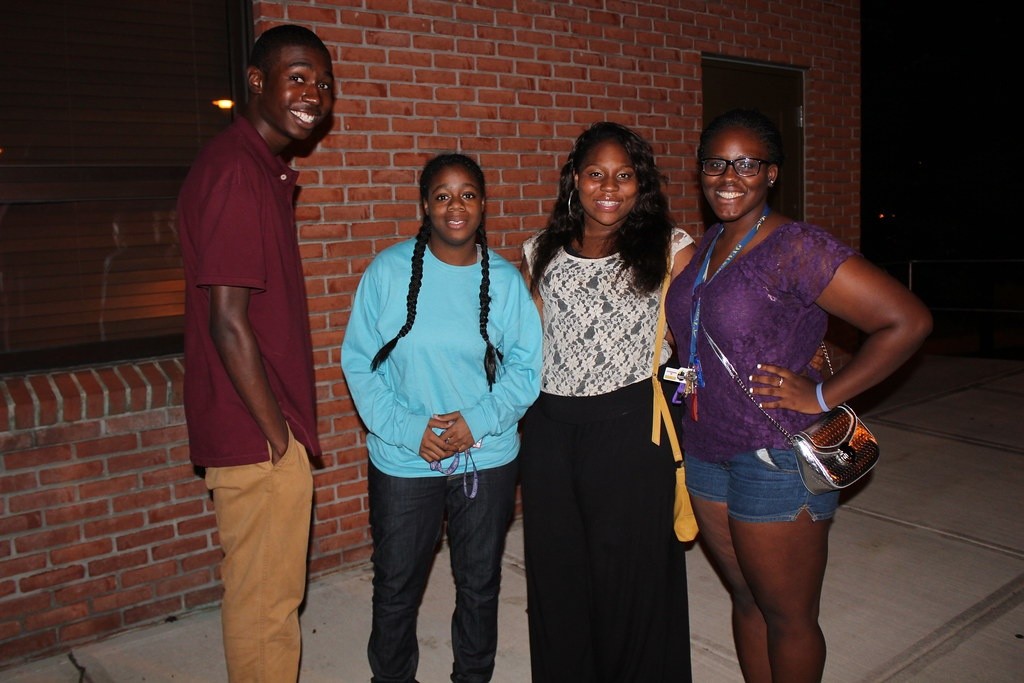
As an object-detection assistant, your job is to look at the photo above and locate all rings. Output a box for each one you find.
[779,377,784,386]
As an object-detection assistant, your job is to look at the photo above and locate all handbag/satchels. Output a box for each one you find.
[675,464,701,541]
[790,403,879,494]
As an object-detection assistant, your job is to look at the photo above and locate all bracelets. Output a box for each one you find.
[816,382,831,412]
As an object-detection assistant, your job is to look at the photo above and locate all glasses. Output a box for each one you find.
[701,156,772,176]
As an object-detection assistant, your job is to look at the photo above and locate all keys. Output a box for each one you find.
[684,371,698,394]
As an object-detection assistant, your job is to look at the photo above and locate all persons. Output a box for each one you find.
[177,25,337,683]
[519,121,833,683]
[341,154,544,683]
[664,111,933,683]
